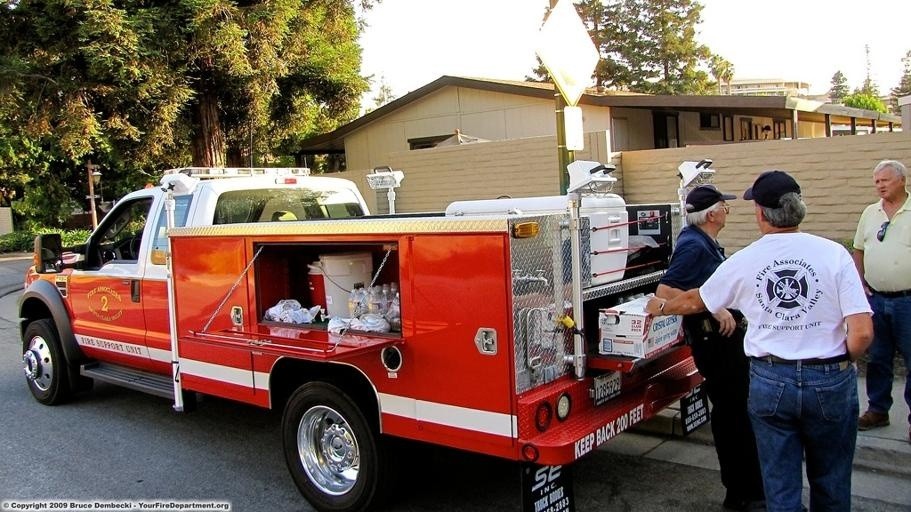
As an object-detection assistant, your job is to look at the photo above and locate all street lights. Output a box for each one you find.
[84,160,101,231]
[88,161,104,229]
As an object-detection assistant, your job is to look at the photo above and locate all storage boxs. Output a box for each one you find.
[598,296,683,359]
[446,193,629,285]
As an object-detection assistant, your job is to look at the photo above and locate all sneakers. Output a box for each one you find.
[857,409,890,430]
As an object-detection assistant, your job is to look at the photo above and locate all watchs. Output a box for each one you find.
[658,300,667,315]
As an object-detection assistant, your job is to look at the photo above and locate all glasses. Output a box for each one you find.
[877,221,890,241]
[716,203,730,214]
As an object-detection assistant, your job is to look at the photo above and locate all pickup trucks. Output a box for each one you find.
[17,156,720,512]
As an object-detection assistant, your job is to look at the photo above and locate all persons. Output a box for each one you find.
[656,185,765,511]
[646,171,874,511]
[853,160,910,443]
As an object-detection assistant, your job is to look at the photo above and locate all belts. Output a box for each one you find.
[869,285,911,299]
[750,354,851,366]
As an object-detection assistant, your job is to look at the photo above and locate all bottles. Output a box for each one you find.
[348,282,397,319]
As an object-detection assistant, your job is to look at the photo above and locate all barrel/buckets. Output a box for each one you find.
[319,252,374,323]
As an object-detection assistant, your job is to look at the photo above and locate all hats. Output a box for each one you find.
[686,185,737,214]
[743,170,801,209]
